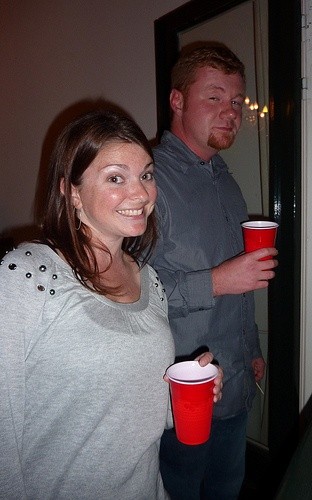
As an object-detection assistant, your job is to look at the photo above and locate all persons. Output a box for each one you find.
[0,112,224,500]
[126,40,278,500]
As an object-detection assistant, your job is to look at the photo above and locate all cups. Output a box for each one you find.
[242,221,279,281]
[166,361,219,446]
